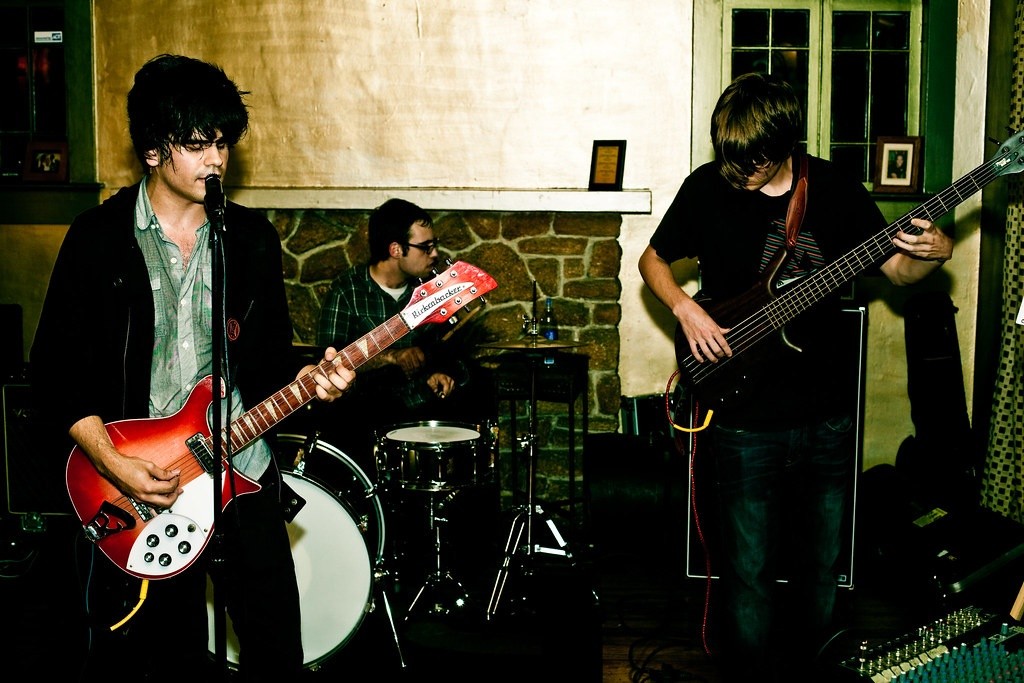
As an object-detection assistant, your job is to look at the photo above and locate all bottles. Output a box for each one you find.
[540,299,557,366]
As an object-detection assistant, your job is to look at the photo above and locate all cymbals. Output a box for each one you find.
[473,333,591,353]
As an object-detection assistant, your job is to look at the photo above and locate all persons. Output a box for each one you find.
[23,54,355,683]
[638,72,952,683]
[888,154,905,178]
[313,198,507,571]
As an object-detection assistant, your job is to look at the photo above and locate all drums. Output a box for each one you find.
[203,429,386,673]
[371,415,498,499]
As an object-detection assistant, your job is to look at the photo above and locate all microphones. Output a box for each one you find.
[205,173,225,233]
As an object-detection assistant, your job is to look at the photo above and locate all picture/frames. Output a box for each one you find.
[588,140,627,191]
[872,136,923,194]
[24,142,69,182]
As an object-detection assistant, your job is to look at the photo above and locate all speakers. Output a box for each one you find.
[684,306,868,585]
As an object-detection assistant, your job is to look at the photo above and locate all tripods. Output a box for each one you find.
[401,356,600,623]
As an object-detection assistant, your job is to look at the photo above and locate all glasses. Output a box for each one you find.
[408,237,437,255]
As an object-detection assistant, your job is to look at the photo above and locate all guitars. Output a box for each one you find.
[674,129,1024,433]
[61,258,501,584]
